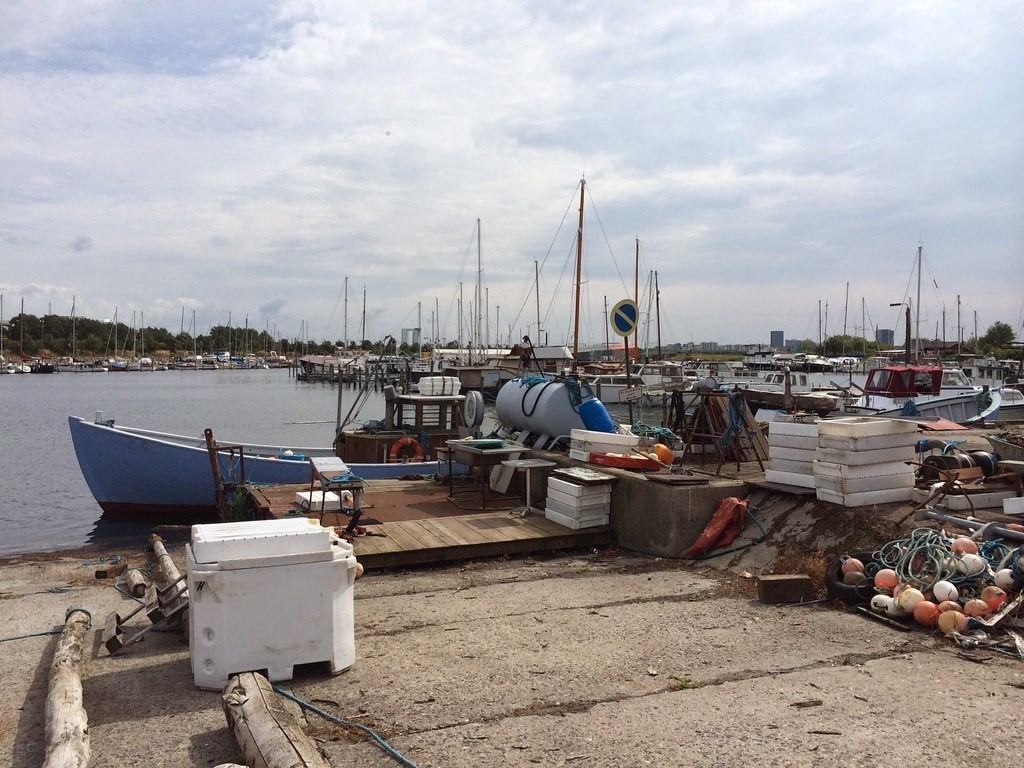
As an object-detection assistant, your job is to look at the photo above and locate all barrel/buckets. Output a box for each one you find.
[279,447,304,461]
[579,398,615,432]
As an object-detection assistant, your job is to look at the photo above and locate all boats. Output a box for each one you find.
[68,333,768,515]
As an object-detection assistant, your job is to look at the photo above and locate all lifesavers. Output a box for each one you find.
[389,438,423,463]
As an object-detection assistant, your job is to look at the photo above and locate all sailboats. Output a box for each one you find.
[298,170,1023,421]
[0,294,297,374]
